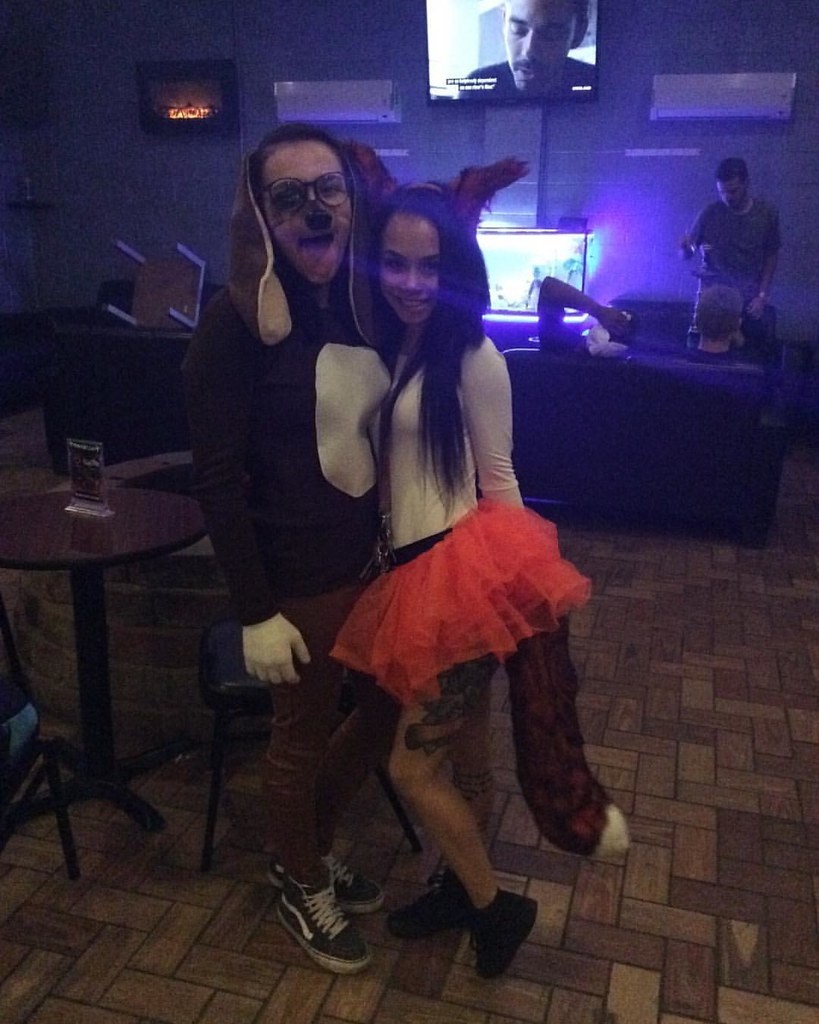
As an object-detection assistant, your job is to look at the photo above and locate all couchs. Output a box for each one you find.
[499,346,785,549]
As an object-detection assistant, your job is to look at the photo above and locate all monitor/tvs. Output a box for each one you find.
[423,0,600,107]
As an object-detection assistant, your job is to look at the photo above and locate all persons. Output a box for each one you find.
[534,276,642,357]
[330,179,541,979]
[676,155,782,349]
[670,282,751,366]
[456,0,596,101]
[181,122,397,979]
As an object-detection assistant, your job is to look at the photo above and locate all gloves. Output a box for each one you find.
[241,611,311,687]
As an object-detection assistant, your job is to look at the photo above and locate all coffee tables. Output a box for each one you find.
[0,485,207,836]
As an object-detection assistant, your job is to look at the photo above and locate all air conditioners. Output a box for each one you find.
[272,78,402,126]
[648,72,799,123]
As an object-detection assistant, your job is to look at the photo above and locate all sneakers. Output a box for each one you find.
[265,850,386,914]
[386,870,478,941]
[476,885,538,979]
[276,869,371,975]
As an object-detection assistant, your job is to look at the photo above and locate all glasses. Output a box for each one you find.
[259,170,352,213]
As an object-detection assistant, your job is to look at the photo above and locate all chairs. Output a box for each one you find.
[198,612,424,878]
[0,591,167,879]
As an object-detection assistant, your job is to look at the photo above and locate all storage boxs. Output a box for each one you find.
[476,216,594,326]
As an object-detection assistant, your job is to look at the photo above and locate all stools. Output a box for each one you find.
[98,233,207,334]
